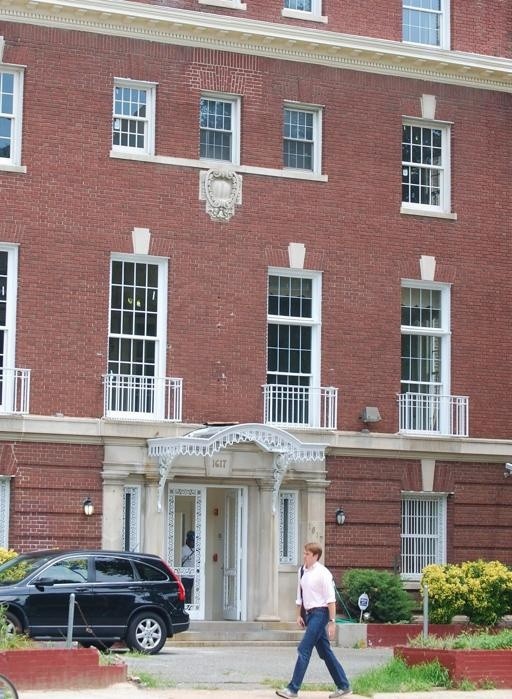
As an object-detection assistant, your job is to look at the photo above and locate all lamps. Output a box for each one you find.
[336,504,347,526]
[83,496,95,517]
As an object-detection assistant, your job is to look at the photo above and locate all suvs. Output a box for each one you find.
[1,547,192,657]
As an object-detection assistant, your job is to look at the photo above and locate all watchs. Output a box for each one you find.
[328,618,335,623]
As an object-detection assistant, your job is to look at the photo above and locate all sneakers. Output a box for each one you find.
[329,689,352,699]
[276,688,298,699]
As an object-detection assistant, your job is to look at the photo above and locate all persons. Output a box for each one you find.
[180,531,195,604]
[275,541,353,698]
